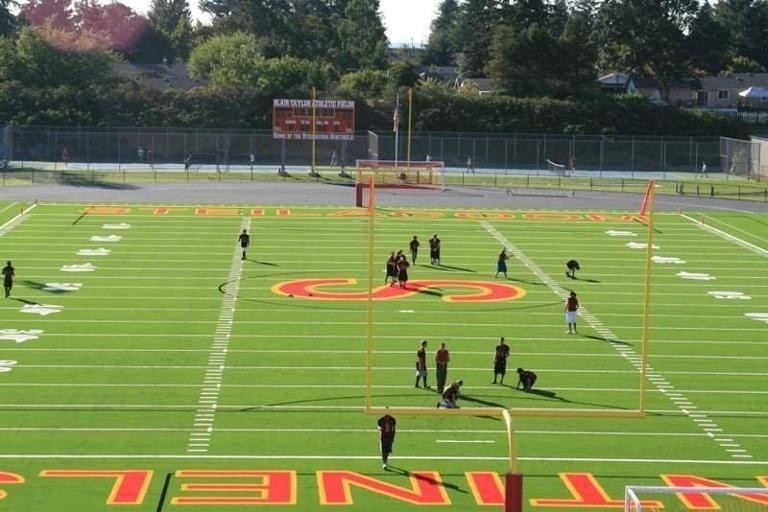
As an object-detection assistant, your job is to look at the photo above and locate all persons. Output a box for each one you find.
[377,409,395,470]
[409,235,419,265]
[238,230,249,261]
[492,337,511,386]
[414,340,429,387]
[700,161,709,177]
[428,234,439,265]
[433,342,451,393]
[425,153,432,169]
[565,292,579,334]
[568,155,576,174]
[436,378,464,408]
[565,259,581,278]
[493,250,513,278]
[249,150,255,169]
[464,156,473,174]
[383,250,409,289]
[2,260,15,298]
[330,151,336,167]
[516,368,538,391]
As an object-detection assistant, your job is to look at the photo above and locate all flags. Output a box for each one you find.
[392,97,398,136]
